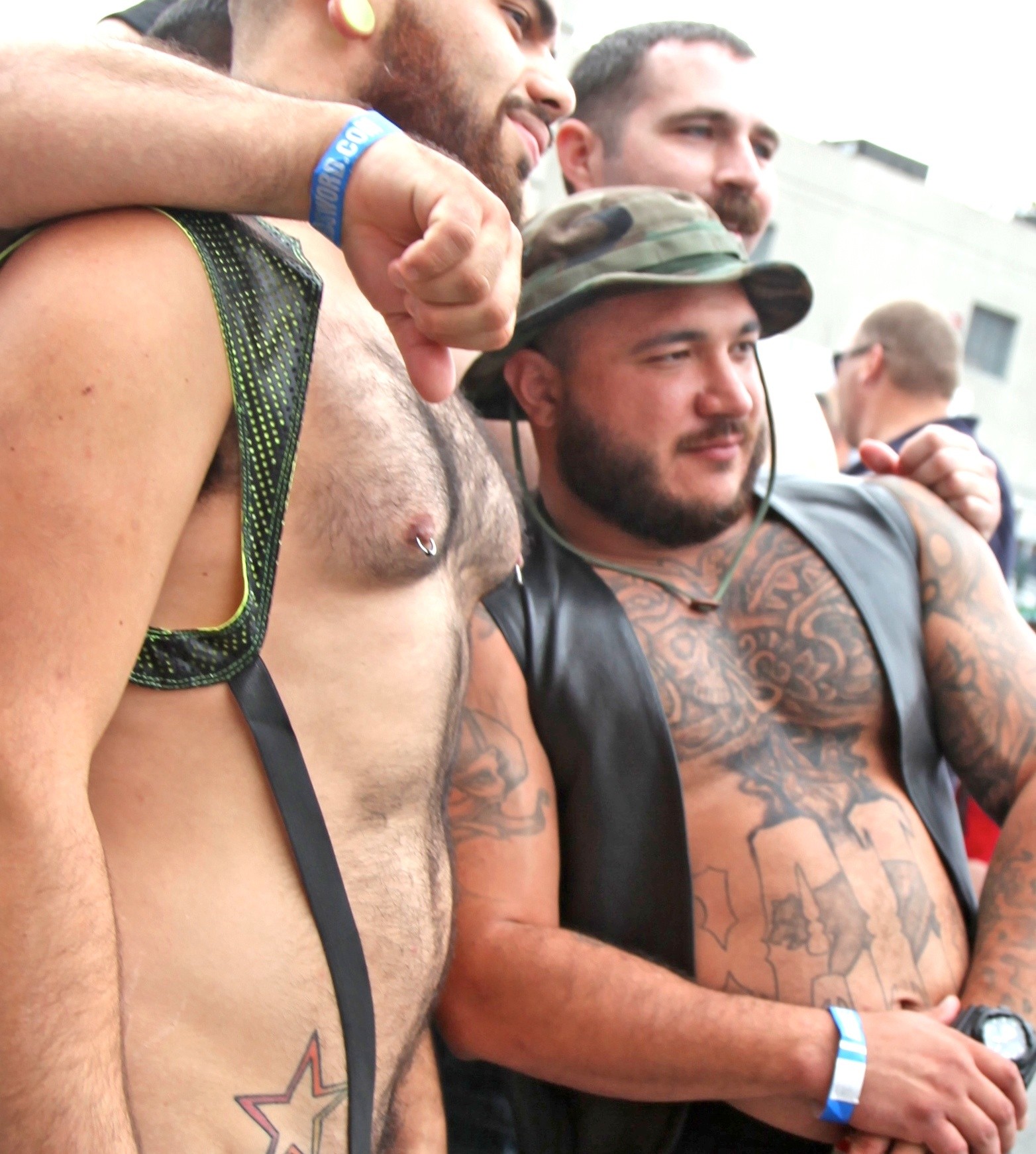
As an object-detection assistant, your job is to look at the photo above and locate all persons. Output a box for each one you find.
[0,0,1036,1154]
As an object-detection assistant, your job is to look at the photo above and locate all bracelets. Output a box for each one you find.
[308,107,399,251]
[818,1005,867,1125]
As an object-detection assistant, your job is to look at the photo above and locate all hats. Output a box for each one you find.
[459,186,813,421]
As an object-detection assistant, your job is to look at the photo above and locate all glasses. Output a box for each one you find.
[832,340,886,377]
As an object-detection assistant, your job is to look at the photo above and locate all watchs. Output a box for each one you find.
[948,1004,1036,1089]
